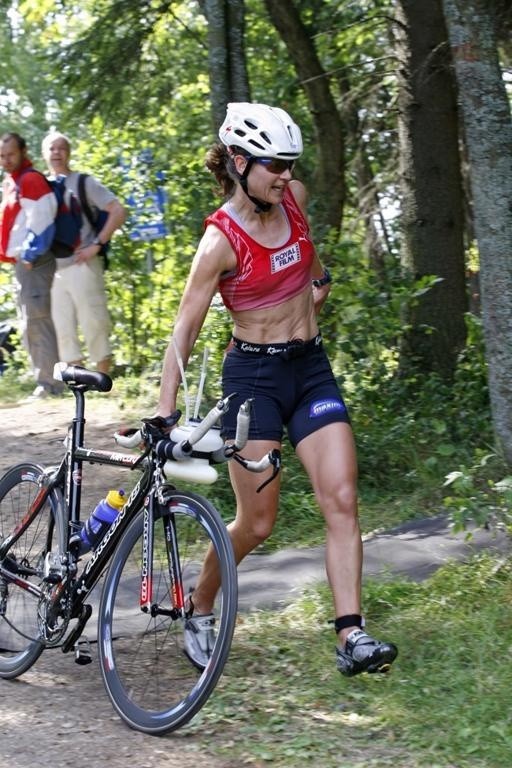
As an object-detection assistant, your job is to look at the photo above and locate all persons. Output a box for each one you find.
[0,131,58,398]
[40,131,127,388]
[149,102,399,678]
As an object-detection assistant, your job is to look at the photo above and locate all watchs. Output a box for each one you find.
[91,238,103,246]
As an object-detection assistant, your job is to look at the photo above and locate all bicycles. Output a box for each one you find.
[0,361,284,737]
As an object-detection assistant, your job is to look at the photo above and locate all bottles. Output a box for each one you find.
[79,489,129,555]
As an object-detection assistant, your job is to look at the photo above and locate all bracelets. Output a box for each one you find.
[313,266,331,287]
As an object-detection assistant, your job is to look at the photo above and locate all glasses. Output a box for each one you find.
[244,154,295,175]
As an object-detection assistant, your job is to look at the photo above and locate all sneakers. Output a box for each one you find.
[181,606,221,674]
[334,629,400,679]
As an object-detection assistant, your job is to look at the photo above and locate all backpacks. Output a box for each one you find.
[78,173,113,256]
[17,168,86,258]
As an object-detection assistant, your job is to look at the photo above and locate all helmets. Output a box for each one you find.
[217,101,305,161]
